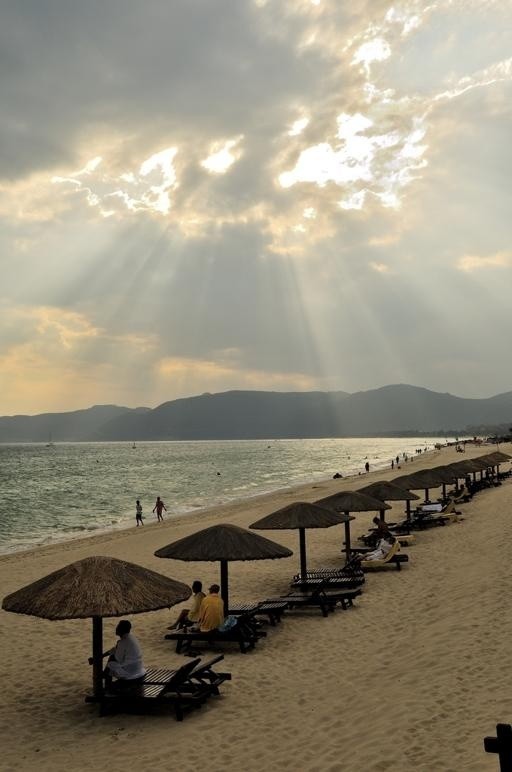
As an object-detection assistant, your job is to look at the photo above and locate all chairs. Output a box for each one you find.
[260,553,409,617]
[86,652,233,718]
[162,603,287,654]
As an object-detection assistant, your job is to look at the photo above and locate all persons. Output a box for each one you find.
[152,497,167,523]
[102,620,146,689]
[365,462,369,472]
[365,517,389,543]
[333,473,343,479]
[364,535,396,562]
[453,484,464,498]
[488,469,494,485]
[166,580,206,630]
[197,584,224,632]
[358,532,392,557]
[135,500,144,526]
